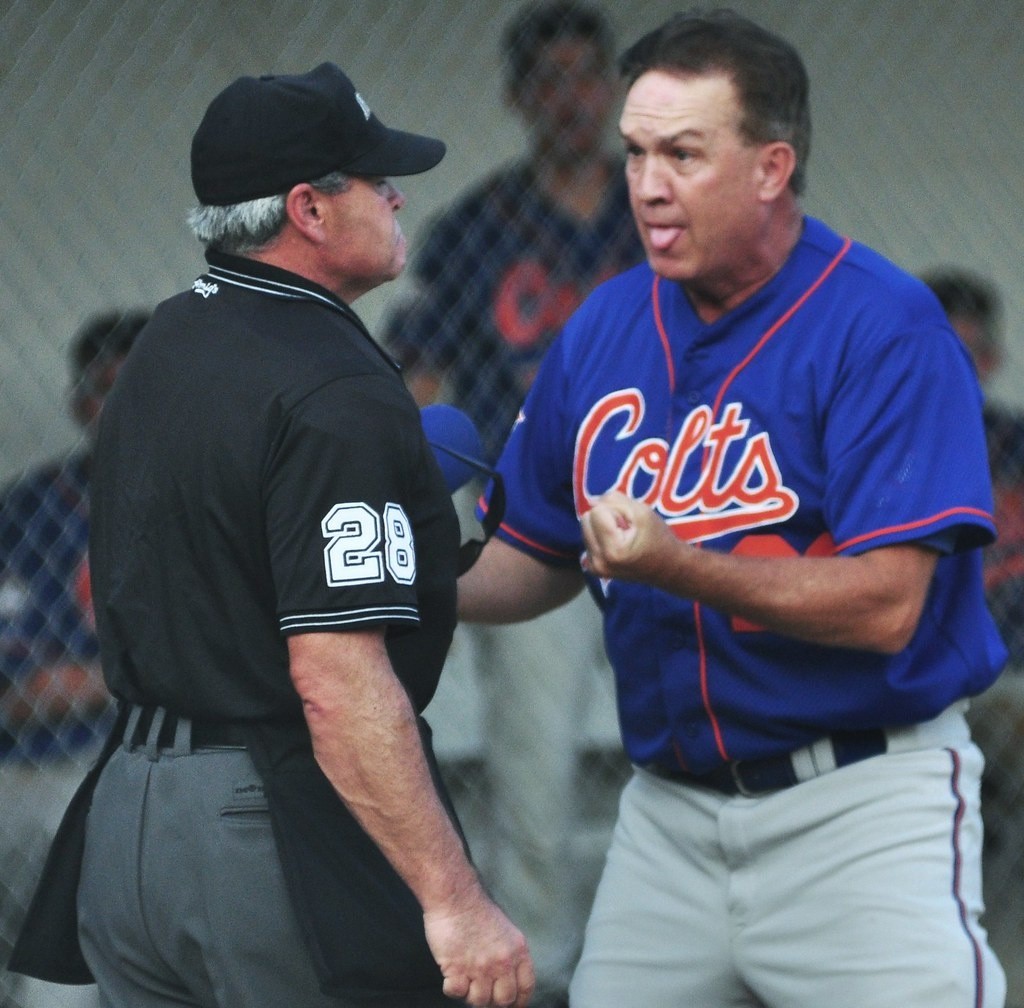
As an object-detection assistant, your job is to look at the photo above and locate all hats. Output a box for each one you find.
[189,62,446,207]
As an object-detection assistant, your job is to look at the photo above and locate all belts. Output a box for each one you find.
[646,729,889,797]
[137,713,250,748]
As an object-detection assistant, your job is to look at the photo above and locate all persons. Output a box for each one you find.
[918,266,1024,1008]
[7,58,535,1008]
[367,0,653,1008]
[0,309,154,1008]
[457,9,1008,1008]
